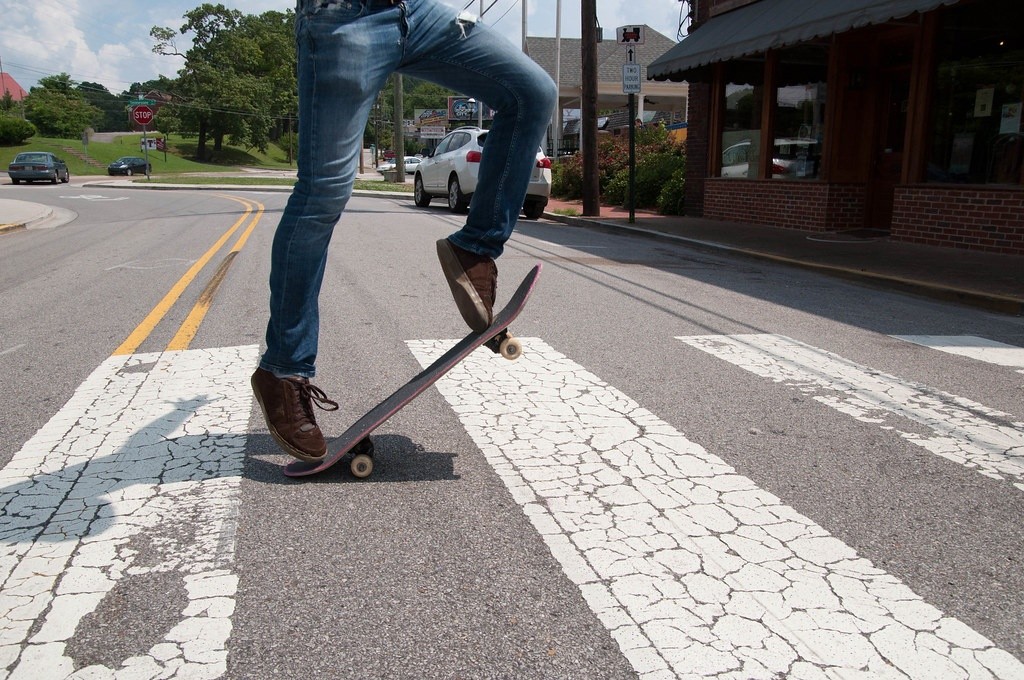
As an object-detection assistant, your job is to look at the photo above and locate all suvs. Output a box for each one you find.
[410,126,553,220]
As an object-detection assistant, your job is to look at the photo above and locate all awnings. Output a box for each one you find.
[647,0,963,84]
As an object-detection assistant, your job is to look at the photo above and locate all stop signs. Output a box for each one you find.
[132,104,155,125]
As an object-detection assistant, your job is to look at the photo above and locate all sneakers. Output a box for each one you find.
[251,367,338,461]
[436,236,498,332]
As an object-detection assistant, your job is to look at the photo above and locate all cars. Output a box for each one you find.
[383,149,399,160]
[7,152,70,185]
[108,156,152,177]
[375,156,424,175]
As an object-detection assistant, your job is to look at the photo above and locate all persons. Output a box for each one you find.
[249,0,558,461]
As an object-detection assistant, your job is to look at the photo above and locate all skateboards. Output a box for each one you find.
[283,264,542,478]
